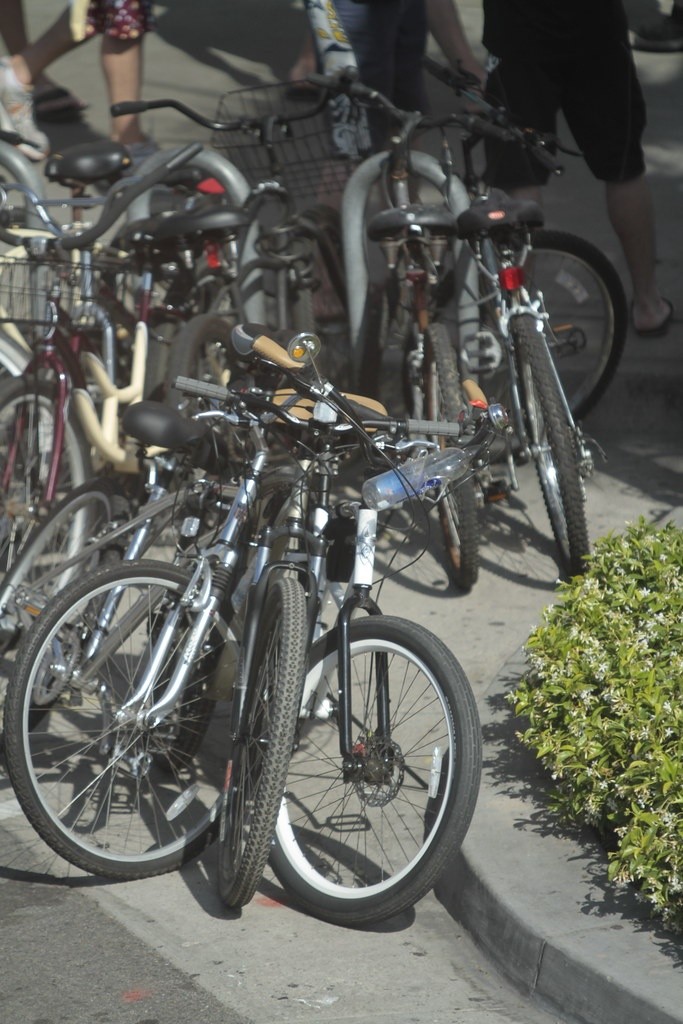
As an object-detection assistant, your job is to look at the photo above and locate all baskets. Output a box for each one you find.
[0,254,129,330]
[208,80,369,196]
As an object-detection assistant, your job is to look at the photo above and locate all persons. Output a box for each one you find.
[292,0,490,316]
[0,0,162,174]
[482,1,677,337]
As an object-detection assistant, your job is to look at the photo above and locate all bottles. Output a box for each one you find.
[362,444,481,512]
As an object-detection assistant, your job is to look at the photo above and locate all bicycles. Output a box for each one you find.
[0,39,622,931]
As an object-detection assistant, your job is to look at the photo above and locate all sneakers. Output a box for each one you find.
[129,139,189,192]
[1,56,50,162]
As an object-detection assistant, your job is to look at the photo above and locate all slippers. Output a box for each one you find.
[632,298,673,335]
[35,88,83,123]
